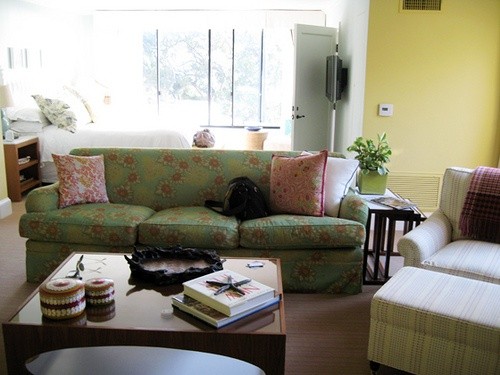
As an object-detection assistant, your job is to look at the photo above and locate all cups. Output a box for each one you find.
[5,130,14,142]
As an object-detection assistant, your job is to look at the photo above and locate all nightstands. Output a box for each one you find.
[3,136,41,202]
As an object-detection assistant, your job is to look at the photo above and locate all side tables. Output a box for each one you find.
[361,194,426,285]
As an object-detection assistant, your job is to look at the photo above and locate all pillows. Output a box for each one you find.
[301,151,360,217]
[270,150,328,217]
[51,153,109,209]
[32,90,94,134]
[10,121,42,133]
[4,96,48,123]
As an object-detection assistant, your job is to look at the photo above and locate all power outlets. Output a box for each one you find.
[379,104,392,116]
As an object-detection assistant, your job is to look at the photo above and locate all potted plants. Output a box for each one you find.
[347,132,392,195]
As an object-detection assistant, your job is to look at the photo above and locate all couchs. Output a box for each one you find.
[19,149,369,294]
[367,167,499,375]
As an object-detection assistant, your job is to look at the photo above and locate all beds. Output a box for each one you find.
[8,80,191,184]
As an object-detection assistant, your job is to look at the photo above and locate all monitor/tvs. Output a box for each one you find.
[326,55,347,103]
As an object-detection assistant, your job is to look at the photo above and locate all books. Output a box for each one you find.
[170,289,283,329]
[371,195,418,211]
[182,268,278,318]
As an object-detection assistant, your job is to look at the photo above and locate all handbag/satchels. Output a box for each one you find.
[204,176,270,219]
